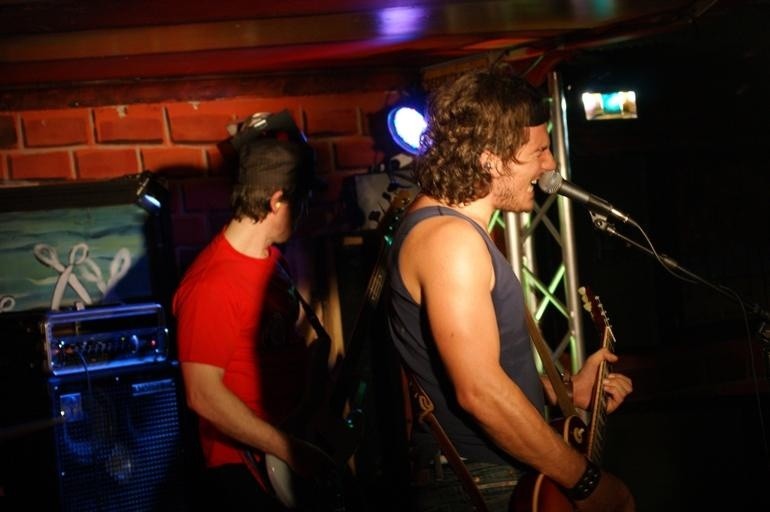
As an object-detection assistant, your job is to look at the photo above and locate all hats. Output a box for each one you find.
[234,134,313,185]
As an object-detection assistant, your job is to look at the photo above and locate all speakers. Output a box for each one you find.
[44,362,188,512]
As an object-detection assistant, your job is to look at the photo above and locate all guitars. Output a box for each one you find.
[258,187,421,511]
[517,292,640,512]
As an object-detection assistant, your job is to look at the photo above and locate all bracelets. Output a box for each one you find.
[555,377,574,408]
[559,455,602,500]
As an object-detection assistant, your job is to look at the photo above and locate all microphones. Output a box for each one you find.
[537,167,629,225]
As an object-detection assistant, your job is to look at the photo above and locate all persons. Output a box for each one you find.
[167,140,331,511]
[380,66,637,511]
